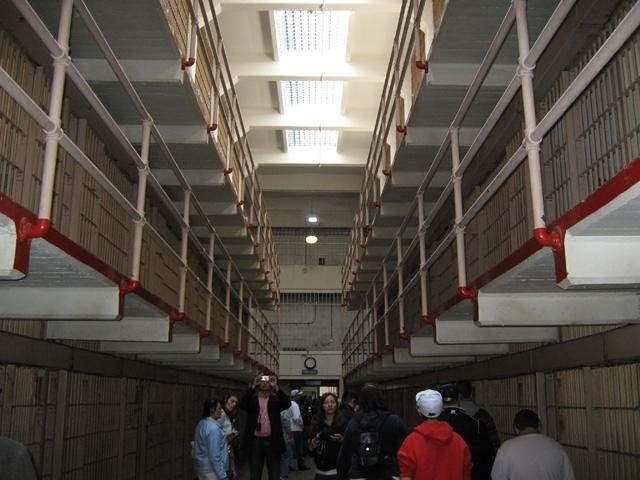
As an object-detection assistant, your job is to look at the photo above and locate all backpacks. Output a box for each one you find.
[348,411,393,480]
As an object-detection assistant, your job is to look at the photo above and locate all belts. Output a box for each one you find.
[252,436,270,440]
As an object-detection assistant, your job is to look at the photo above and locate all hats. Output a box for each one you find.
[291,390,303,396]
[437,382,459,403]
[415,389,444,418]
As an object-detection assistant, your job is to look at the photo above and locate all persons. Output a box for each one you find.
[490,409,571,477]
[194,374,500,478]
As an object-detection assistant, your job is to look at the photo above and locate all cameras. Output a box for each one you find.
[260,375,269,382]
[319,431,329,441]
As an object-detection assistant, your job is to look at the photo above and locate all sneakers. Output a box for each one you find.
[301,467,311,471]
